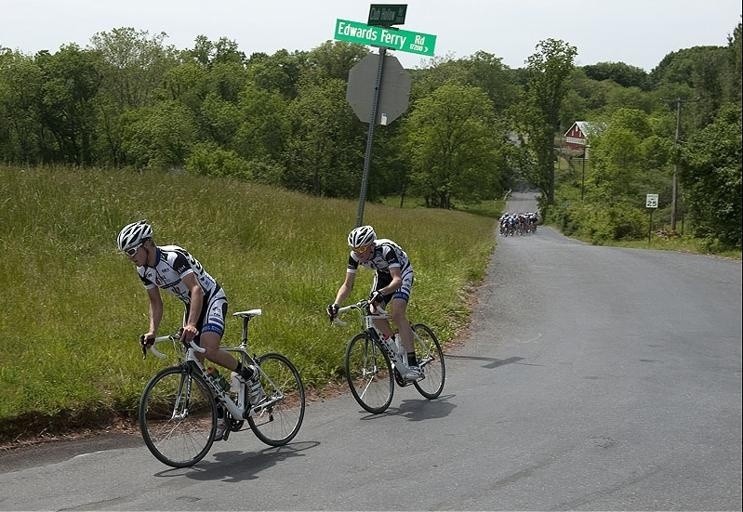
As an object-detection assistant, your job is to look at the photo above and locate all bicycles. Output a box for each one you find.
[326,292,447,415]
[499,219,537,237]
[136,307,305,468]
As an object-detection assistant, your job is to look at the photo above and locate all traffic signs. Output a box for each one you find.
[334,18,436,57]
[646,194,659,207]
[367,3,407,26]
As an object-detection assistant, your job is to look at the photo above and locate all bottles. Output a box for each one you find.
[206,366,233,394]
[382,334,398,354]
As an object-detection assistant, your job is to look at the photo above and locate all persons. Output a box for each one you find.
[326,225,422,379]
[498,210,540,237]
[116,220,262,442]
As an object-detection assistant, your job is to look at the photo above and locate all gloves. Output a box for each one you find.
[326,304,339,319]
[366,289,385,307]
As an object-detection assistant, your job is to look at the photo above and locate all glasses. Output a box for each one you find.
[125,238,149,258]
[351,245,373,255]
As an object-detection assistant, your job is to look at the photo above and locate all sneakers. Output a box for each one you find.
[242,364,264,406]
[207,411,232,441]
[404,364,423,381]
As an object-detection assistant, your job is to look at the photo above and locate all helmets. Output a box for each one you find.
[503,211,539,226]
[346,225,377,248]
[116,219,154,252]
